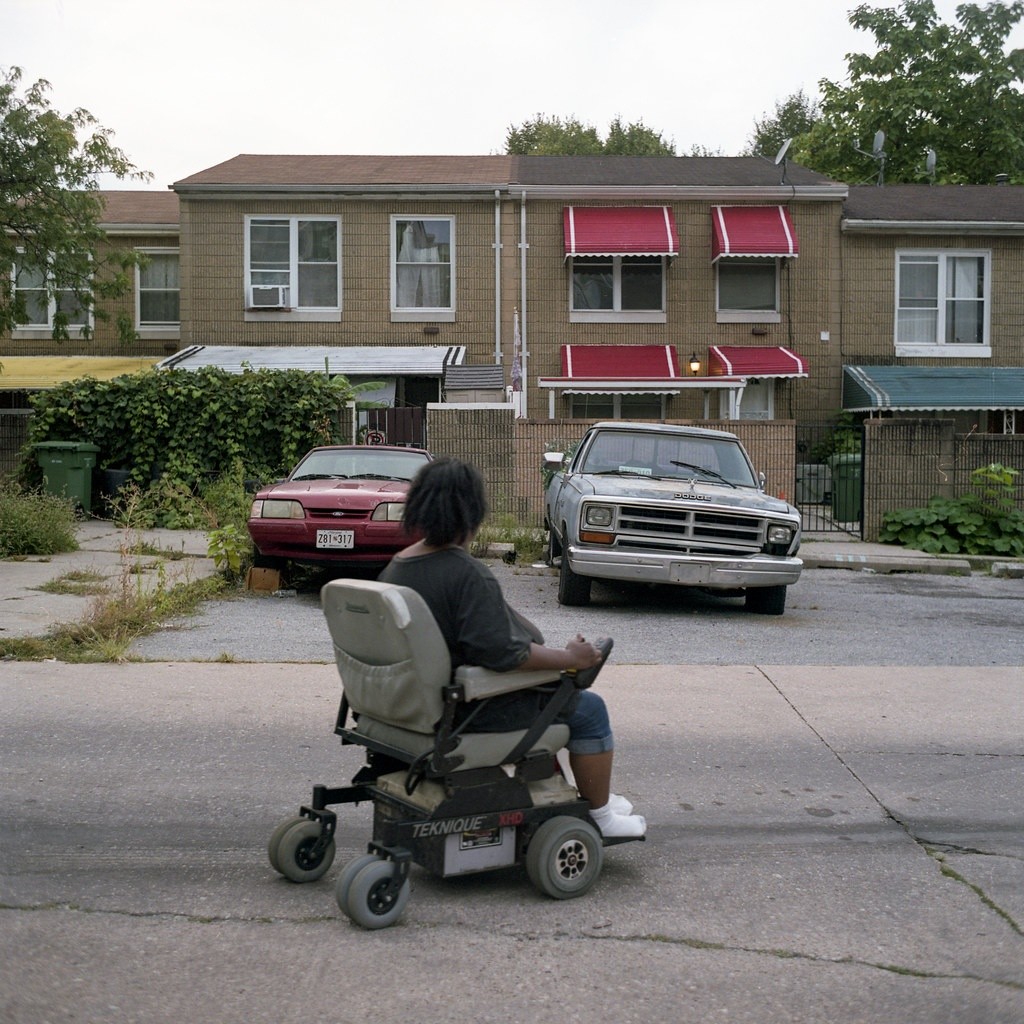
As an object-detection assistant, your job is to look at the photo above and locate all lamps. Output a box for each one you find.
[690,352,700,376]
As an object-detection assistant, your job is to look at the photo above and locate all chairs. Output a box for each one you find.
[320,578,580,772]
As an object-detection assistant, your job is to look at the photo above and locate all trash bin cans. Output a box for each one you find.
[32,440,101,522]
[827,453,861,523]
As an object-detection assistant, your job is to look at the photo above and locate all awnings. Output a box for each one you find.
[559,343,681,395]
[708,346,808,379]
[710,205,799,264]
[156,345,467,375]
[563,205,679,264]
[841,365,1024,411]
[0,356,169,394]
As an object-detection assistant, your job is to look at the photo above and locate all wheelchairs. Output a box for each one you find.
[266,579,651,934]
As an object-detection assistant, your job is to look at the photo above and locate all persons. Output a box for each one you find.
[377,458,647,836]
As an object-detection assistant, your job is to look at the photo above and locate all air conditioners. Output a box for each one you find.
[251,285,286,308]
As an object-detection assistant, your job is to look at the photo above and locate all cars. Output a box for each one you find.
[541,423,803,618]
[245,447,441,595]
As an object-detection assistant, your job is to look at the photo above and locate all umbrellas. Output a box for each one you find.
[510,306,522,391]
